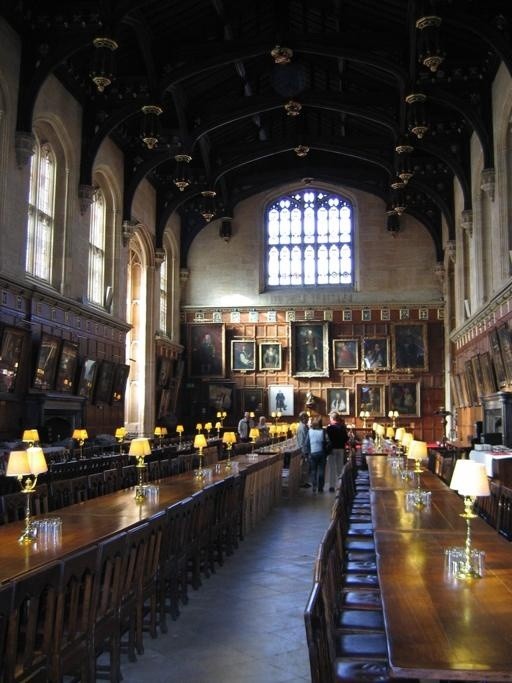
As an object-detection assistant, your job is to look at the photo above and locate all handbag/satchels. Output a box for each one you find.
[322,429,333,456]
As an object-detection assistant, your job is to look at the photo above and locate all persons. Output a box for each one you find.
[304,415,333,496]
[336,416,346,433]
[296,411,312,487]
[326,412,348,492]
[156,360,175,393]
[236,411,256,442]
[257,415,270,441]
[190,323,426,418]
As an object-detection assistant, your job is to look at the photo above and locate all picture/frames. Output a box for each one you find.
[0,321,132,409]
[156,317,431,424]
[442,321,512,409]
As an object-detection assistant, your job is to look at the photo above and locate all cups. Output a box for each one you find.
[445,546,487,577]
[32,515,63,532]
[144,484,160,495]
[403,488,432,504]
